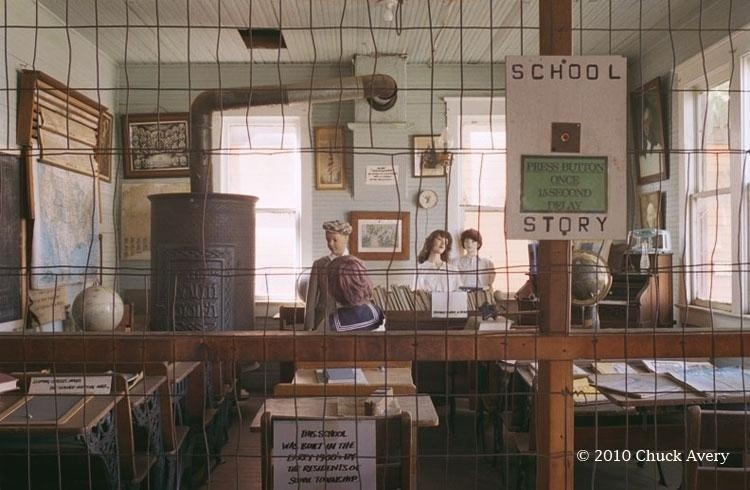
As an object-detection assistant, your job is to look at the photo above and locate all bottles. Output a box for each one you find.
[639,242,651,272]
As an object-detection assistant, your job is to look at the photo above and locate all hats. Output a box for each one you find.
[324,221,352,235]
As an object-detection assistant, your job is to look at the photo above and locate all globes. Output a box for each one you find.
[72,285,124,332]
[571,250,613,329]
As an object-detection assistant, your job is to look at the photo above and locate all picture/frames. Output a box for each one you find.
[639,191,663,229]
[121,112,189,179]
[631,75,668,184]
[313,126,346,190]
[351,210,410,260]
[412,134,447,177]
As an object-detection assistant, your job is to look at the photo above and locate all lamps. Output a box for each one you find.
[378,0,398,21]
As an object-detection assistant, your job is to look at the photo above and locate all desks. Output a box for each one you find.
[278,304,541,330]
[250,359,439,490]
[452,360,750,490]
[0,362,233,490]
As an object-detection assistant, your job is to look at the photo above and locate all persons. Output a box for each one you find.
[411,228,463,292]
[635,105,665,177]
[312,254,387,333]
[454,227,497,288]
[302,219,366,332]
[643,198,661,229]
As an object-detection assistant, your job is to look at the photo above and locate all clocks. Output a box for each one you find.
[419,189,438,208]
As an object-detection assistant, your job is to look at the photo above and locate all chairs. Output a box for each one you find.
[63,302,134,332]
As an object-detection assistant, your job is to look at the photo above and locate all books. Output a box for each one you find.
[368,283,516,332]
[0,371,21,395]
[315,367,369,384]
[325,367,355,383]
[502,357,749,409]
[335,396,402,417]
[127,369,144,391]
[106,368,139,382]
